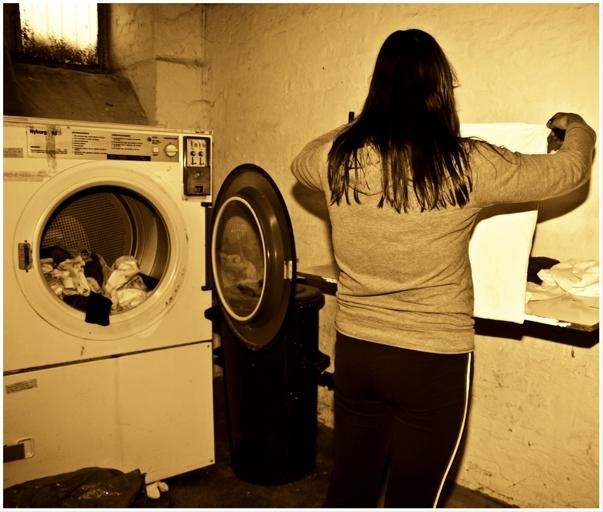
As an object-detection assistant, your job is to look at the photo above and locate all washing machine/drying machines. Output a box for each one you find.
[3,117,300,490]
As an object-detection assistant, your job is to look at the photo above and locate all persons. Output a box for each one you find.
[290,28,596,508]
